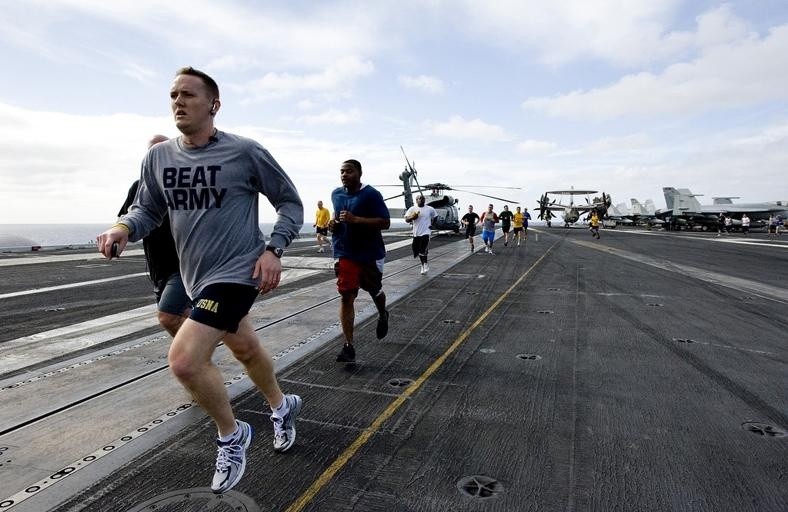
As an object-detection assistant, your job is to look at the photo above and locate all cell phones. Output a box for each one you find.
[112,242,119,257]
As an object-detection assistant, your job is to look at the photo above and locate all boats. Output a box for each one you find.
[1,218,788,512]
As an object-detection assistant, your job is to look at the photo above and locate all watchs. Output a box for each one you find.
[264,243,285,260]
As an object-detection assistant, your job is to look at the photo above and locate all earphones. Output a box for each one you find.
[212,104,216,110]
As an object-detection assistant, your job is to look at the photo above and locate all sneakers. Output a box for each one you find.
[210,419,251,494]
[592,232,600,239]
[485,246,492,254]
[336,343,355,362]
[330,242,333,249]
[421,263,429,274]
[470,238,474,252]
[316,248,324,252]
[270,394,302,451]
[377,311,388,338]
[504,236,527,247]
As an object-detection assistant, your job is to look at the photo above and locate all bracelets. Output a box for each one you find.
[113,223,131,235]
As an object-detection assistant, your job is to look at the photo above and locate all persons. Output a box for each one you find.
[461,205,481,252]
[313,200,333,253]
[480,203,500,256]
[511,207,524,248]
[646,212,786,237]
[328,160,392,364]
[98,65,302,496]
[522,208,531,235]
[404,194,439,276]
[589,210,600,239]
[497,205,513,245]
[121,135,192,339]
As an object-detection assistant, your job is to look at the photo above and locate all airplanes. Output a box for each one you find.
[532,186,610,227]
[603,186,787,231]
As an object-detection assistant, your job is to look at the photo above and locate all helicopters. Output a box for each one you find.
[370,142,522,235]
